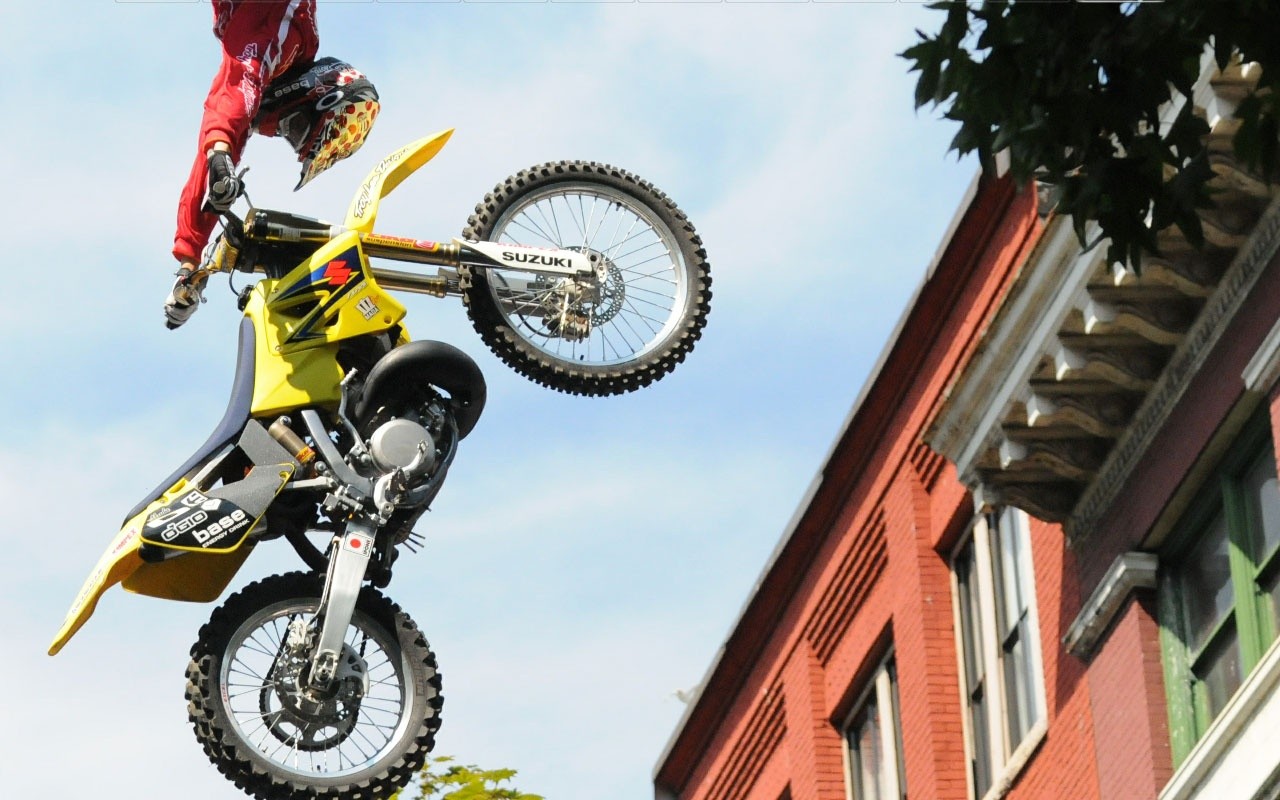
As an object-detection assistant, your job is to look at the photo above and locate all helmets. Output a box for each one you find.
[253,56,381,192]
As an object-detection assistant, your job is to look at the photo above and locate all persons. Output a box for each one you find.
[165,1,380,332]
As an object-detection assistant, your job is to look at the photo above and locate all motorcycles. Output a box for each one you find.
[47,125,711,800]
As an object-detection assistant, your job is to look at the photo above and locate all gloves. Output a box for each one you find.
[200,150,242,217]
[163,266,200,328]
